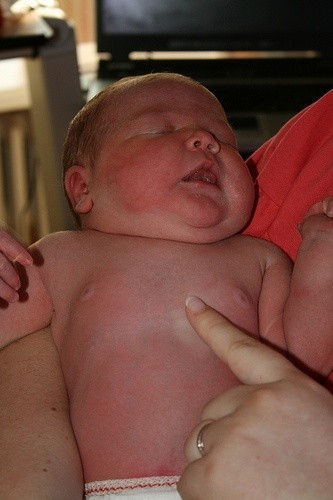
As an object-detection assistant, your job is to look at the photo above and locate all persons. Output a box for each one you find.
[0,72,333,500]
[0,87,333,500]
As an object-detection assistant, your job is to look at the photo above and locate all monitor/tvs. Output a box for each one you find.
[94,0,333,81]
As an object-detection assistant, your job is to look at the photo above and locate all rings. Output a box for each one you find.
[197,421,213,457]
